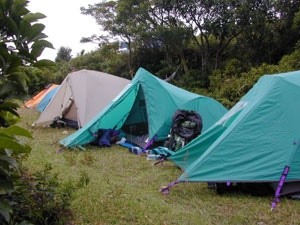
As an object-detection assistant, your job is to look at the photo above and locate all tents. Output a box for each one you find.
[55,66,229,154]
[154,67,300,214]
[26,69,131,131]
[23,84,61,113]
